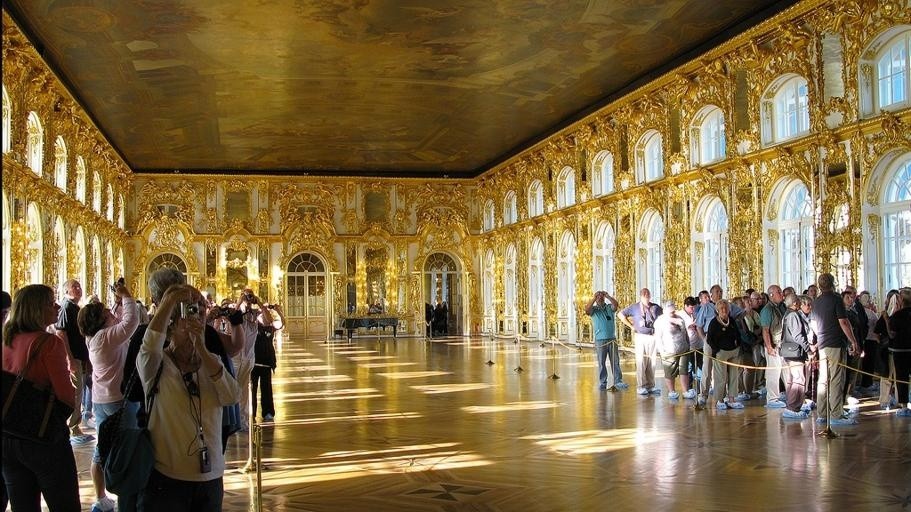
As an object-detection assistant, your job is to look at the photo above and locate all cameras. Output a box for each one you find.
[268,305,274,309]
[246,292,252,300]
[220,308,230,315]
[181,299,202,318]
[109,278,127,291]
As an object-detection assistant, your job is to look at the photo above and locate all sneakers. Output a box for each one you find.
[696,395,706,405]
[716,401,743,409]
[852,382,879,397]
[816,410,855,425]
[91,498,118,511]
[767,391,786,407]
[599,384,606,390]
[668,389,695,399]
[734,388,766,400]
[638,386,660,394]
[782,400,815,418]
[70,433,92,444]
[614,383,628,389]
[881,394,911,416]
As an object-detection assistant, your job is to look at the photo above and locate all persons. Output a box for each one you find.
[425,301,449,335]
[585,272,911,424]
[2,269,286,512]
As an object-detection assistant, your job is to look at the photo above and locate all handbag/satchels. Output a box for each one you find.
[99,409,155,496]
[0,369,74,445]
[779,342,801,359]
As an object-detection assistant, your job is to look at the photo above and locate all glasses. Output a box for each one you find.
[182,371,201,398]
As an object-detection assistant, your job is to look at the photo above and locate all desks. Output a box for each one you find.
[340,315,398,338]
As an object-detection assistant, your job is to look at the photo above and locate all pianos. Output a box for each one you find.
[340,318,399,345]
